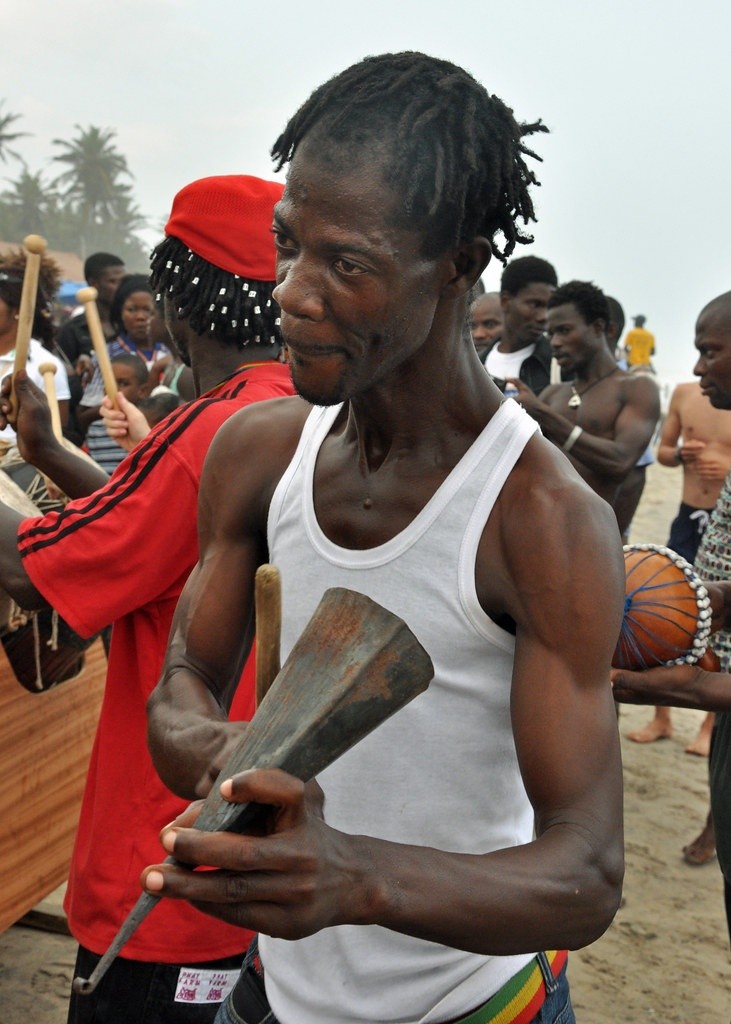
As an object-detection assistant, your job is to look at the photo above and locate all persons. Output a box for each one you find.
[0,244,728,947]
[141,47,625,1024]
[0,173,284,1024]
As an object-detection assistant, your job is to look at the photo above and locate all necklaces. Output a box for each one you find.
[567,366,621,410]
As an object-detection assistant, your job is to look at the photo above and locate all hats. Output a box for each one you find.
[164,175,285,282]
[633,315,646,322]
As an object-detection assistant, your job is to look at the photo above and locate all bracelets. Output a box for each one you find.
[675,445,684,465]
